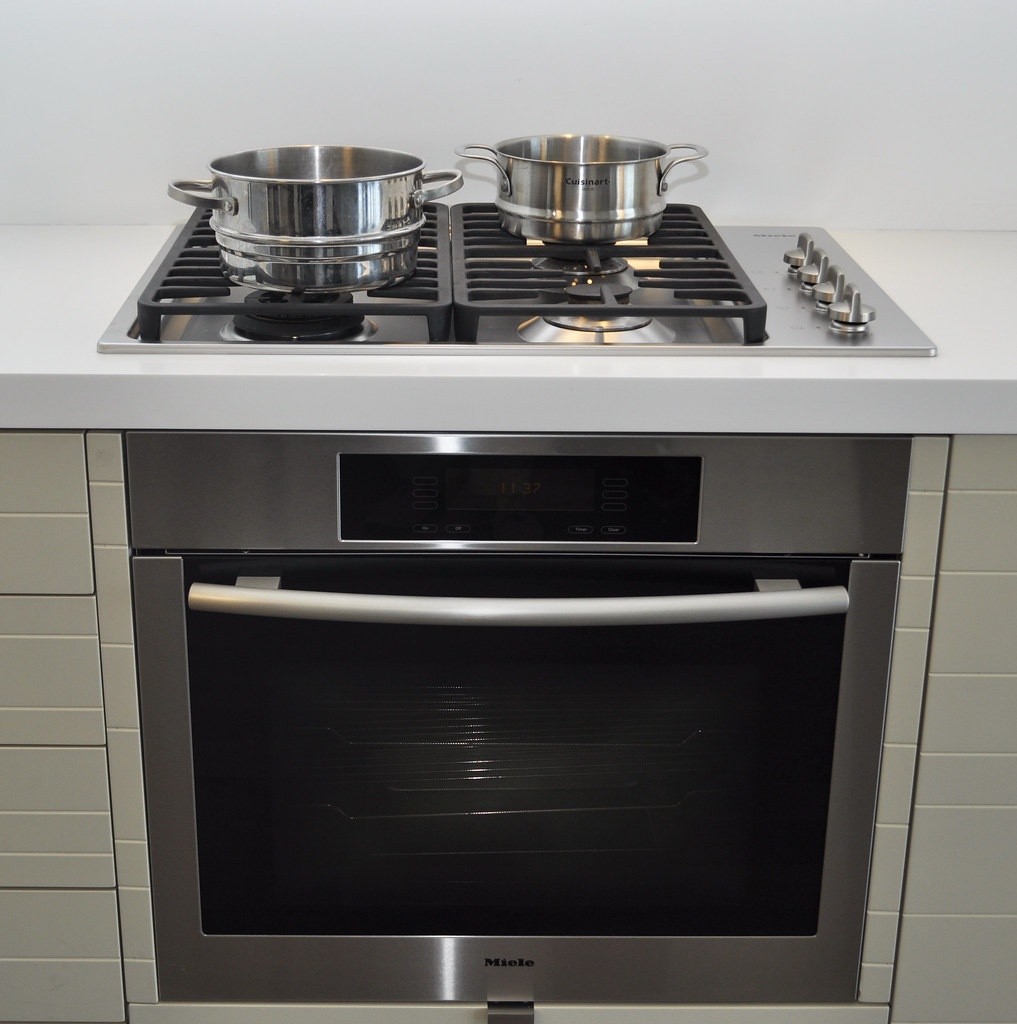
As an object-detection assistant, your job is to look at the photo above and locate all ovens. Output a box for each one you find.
[124,429,914,1024]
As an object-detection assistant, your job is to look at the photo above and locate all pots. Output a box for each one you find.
[166,145,465,294]
[454,134,708,244]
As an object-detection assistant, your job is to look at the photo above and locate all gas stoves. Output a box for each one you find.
[96,203,937,358]
